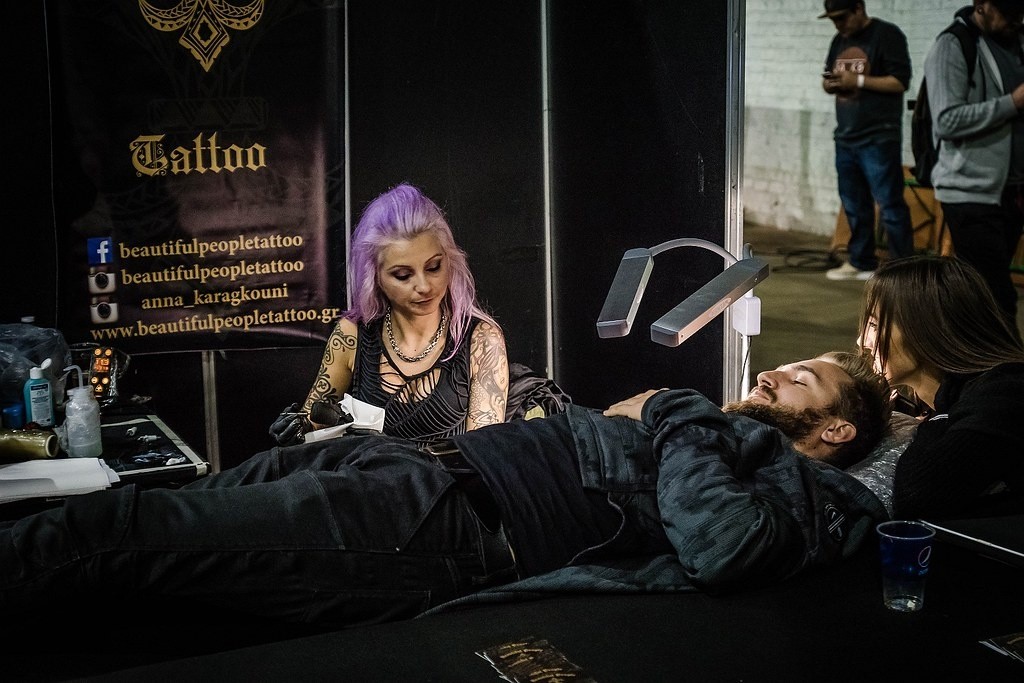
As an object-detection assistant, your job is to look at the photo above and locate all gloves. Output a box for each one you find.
[268,403,314,447]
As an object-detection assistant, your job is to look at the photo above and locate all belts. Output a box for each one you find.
[422,438,518,589]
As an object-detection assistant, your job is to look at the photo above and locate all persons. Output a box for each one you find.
[816,0,915,283]
[853,252,1024,525]
[0,350,895,667]
[267,183,511,450]
[928,0,1023,327]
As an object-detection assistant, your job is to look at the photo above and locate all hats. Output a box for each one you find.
[816,0,864,19]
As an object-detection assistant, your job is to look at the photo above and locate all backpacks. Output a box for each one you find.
[906,20,977,185]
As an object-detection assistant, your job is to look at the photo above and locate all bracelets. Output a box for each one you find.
[856,74,866,89]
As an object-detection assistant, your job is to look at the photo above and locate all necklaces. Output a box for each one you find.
[384,304,447,361]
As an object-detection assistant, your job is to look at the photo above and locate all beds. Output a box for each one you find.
[99,406,964,682]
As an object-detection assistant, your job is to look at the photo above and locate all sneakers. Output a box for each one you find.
[825,260,875,282]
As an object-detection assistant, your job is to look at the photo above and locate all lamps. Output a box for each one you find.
[595,236,772,404]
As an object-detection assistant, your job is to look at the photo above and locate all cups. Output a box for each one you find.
[876,519,936,612]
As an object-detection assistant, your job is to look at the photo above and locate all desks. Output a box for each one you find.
[0,411,211,502]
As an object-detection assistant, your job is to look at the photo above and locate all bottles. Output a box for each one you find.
[67,385,103,458]
